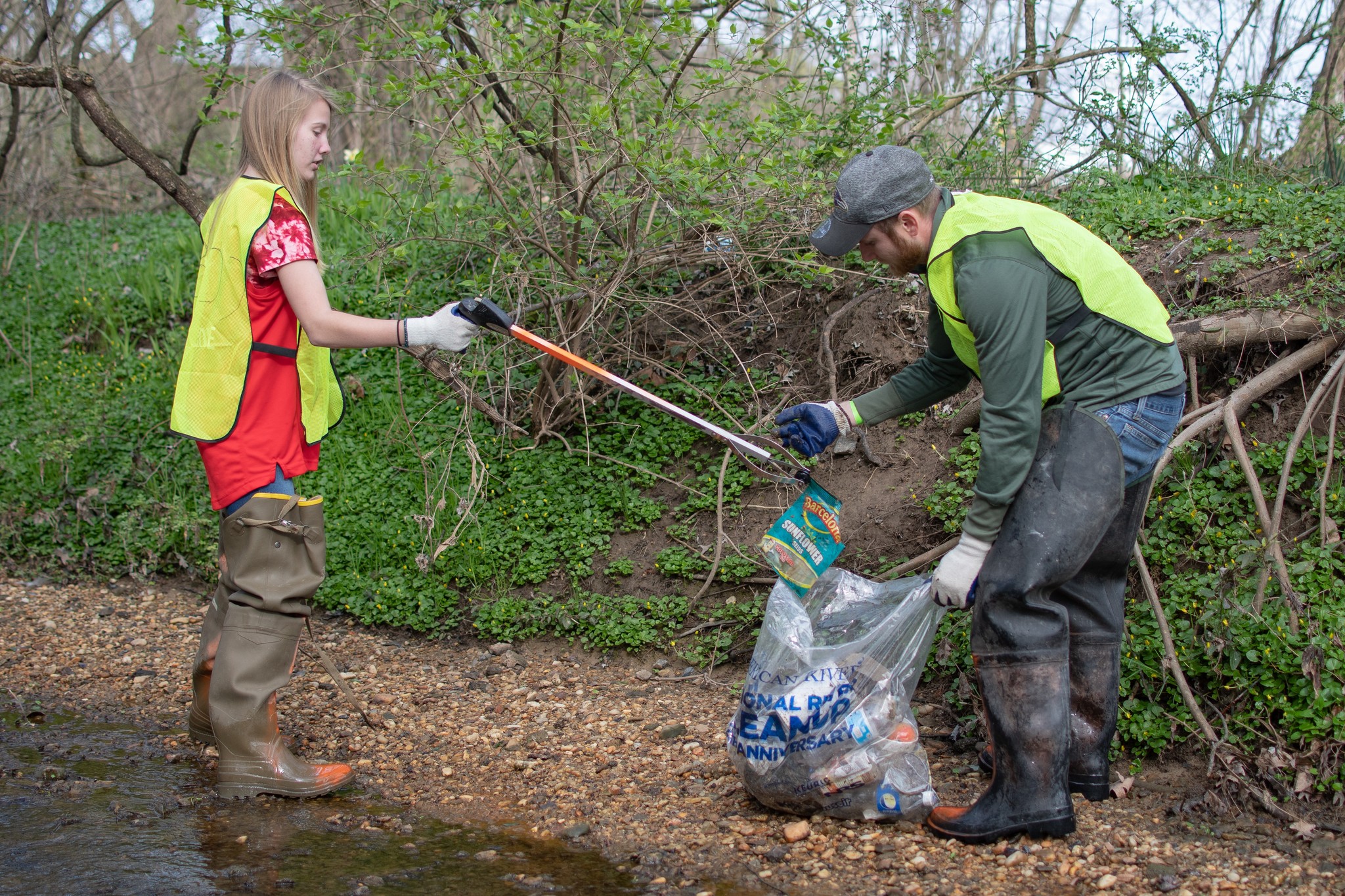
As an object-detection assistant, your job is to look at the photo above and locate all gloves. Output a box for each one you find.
[771,401,852,457]
[923,530,992,612]
[404,301,480,352]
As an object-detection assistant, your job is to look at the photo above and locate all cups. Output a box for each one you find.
[774,660,859,737]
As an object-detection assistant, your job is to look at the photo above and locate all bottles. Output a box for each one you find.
[795,696,935,821]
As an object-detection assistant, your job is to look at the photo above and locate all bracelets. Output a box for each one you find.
[397,320,402,346]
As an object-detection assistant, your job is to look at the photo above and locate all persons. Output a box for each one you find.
[774,145,1188,844]
[169,70,479,799]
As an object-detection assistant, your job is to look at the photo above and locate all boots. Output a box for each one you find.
[926,401,1127,845]
[208,492,356,799]
[188,510,299,755]
[979,459,1156,801]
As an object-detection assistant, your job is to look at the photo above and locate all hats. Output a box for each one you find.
[808,145,934,257]
